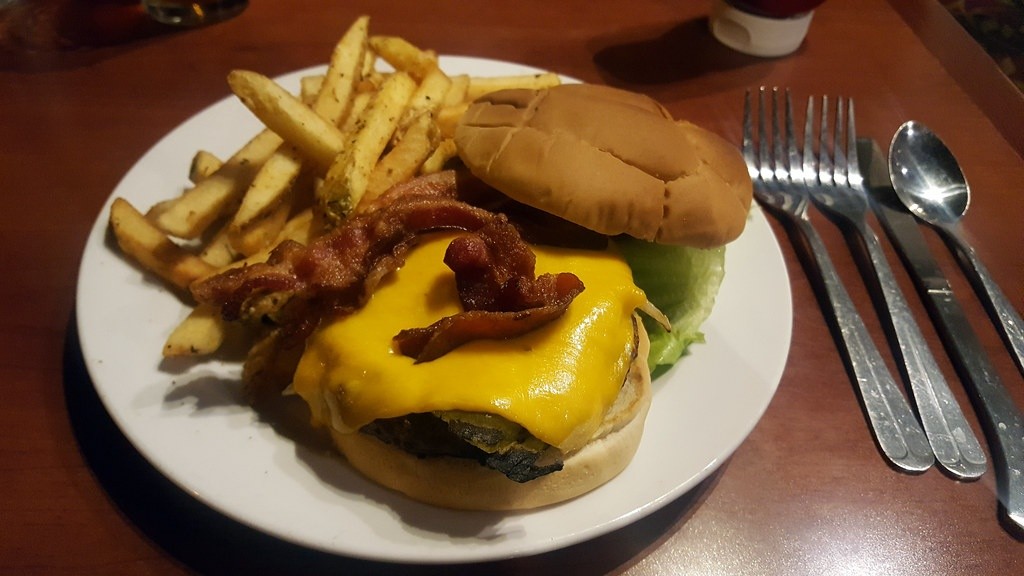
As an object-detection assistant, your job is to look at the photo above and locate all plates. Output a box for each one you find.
[76,53,793,563]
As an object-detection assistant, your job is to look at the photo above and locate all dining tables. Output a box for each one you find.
[0,0,1023,576]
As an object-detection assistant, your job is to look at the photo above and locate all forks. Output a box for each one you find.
[740,82,935,475]
[801,91,993,484]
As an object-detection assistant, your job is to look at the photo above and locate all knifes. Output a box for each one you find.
[850,138,1024,531]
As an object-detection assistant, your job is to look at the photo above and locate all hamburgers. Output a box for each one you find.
[291,84,751,513]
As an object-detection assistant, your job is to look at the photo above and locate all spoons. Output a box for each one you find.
[891,120,1024,382]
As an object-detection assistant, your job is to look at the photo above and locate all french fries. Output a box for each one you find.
[111,17,562,360]
